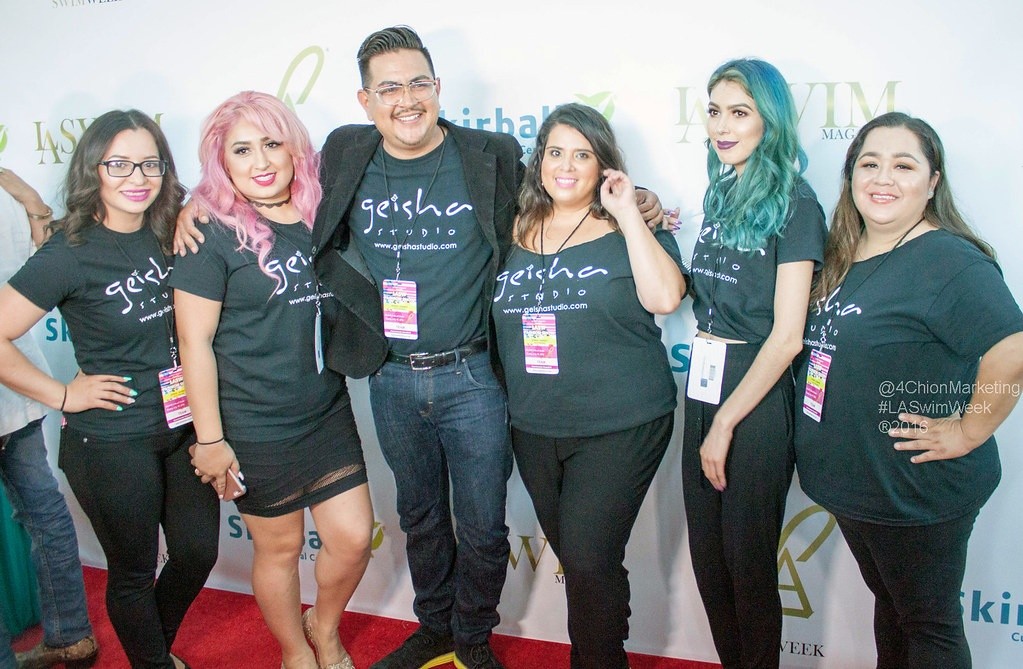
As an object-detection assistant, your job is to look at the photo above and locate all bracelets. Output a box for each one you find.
[196,438,225,446]
[60,384,68,412]
[27,205,54,220]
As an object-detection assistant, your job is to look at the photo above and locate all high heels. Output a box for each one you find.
[301,608,355,669]
[15,636,99,668]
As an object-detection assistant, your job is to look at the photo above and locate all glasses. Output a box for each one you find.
[364,82,437,105]
[96,160,167,177]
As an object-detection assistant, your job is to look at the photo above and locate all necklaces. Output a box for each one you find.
[246,195,292,210]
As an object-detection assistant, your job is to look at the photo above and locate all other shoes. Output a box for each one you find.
[170,654,190,669]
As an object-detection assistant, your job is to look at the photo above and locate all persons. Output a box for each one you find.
[1,110,221,669]
[169,25,691,669]
[662,58,826,669]
[795,111,1023,669]
[0,163,102,663]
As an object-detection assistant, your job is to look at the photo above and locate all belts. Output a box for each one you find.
[386,339,486,370]
[698,330,747,344]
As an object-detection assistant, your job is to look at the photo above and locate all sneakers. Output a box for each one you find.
[368,627,454,669]
[453,642,504,669]
